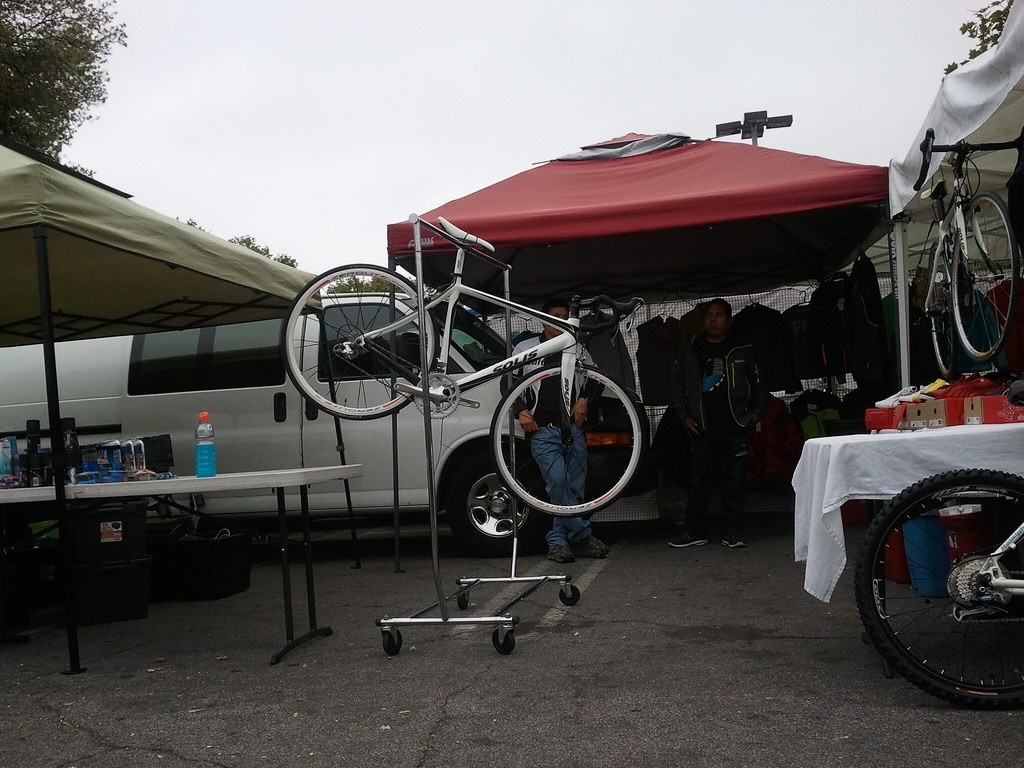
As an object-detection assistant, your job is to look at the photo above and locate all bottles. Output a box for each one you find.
[195,411,216,478]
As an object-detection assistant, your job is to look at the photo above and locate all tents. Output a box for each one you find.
[384,131,897,579]
[891,0,1024,420]
[1,143,365,676]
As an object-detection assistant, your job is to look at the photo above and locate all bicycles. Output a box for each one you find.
[904,129,1024,382]
[276,210,652,515]
[853,374,1024,709]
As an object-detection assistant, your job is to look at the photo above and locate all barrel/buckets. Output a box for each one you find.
[887,510,992,598]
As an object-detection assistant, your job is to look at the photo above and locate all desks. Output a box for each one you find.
[2,464,364,664]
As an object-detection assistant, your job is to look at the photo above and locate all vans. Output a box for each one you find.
[0,302,562,558]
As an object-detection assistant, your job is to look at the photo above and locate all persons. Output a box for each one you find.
[499,299,613,563]
[665,297,751,549]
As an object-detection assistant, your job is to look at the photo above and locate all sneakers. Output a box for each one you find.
[547,545,575,562]
[577,537,609,558]
[668,532,708,547]
[722,534,746,547]
